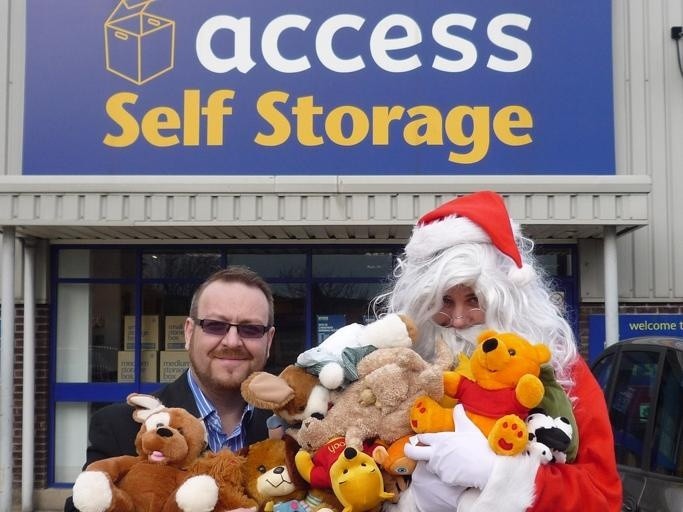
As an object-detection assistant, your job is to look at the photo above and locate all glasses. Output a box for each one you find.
[432,308,485,328]
[195,319,269,338]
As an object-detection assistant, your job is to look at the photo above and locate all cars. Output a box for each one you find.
[588,337,683,511]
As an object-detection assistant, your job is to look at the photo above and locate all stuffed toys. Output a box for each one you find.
[71,392,221,512]
[186,313,579,512]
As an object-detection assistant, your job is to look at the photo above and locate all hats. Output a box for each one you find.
[405,191,522,268]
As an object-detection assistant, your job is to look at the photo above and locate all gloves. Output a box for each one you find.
[404,404,497,491]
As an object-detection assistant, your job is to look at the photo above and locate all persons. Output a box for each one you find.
[295,188,624,512]
[64,259,276,512]
